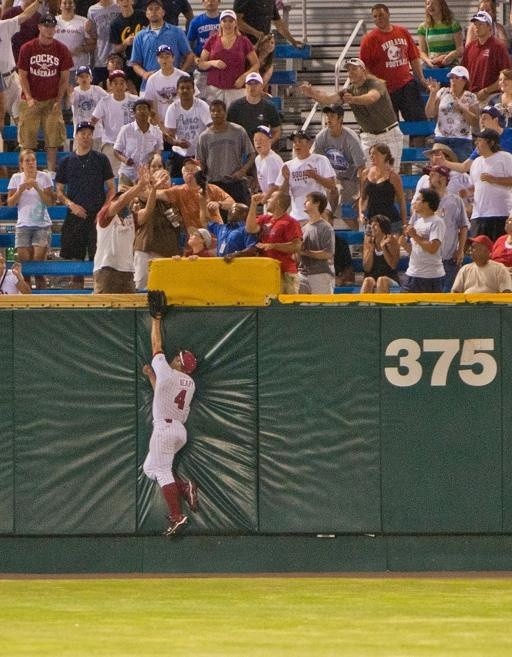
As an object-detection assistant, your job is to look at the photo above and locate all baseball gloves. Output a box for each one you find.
[147,290,173,319]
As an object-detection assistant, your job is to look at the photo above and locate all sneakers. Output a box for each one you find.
[163,515,187,535]
[182,481,198,511]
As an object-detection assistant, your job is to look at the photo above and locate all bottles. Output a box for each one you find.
[6,242,15,262]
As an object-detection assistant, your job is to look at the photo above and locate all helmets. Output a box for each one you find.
[178,351,197,374]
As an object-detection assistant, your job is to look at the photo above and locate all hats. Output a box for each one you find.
[38,14,56,25]
[469,235,493,250]
[183,158,201,165]
[187,226,211,248]
[245,72,263,84]
[424,66,500,185]
[471,11,492,25]
[75,45,173,81]
[220,10,237,20]
[341,58,365,69]
[250,104,343,140]
[77,121,94,131]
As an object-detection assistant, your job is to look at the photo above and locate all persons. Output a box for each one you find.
[0,3,511,299]
[141,289,202,537]
